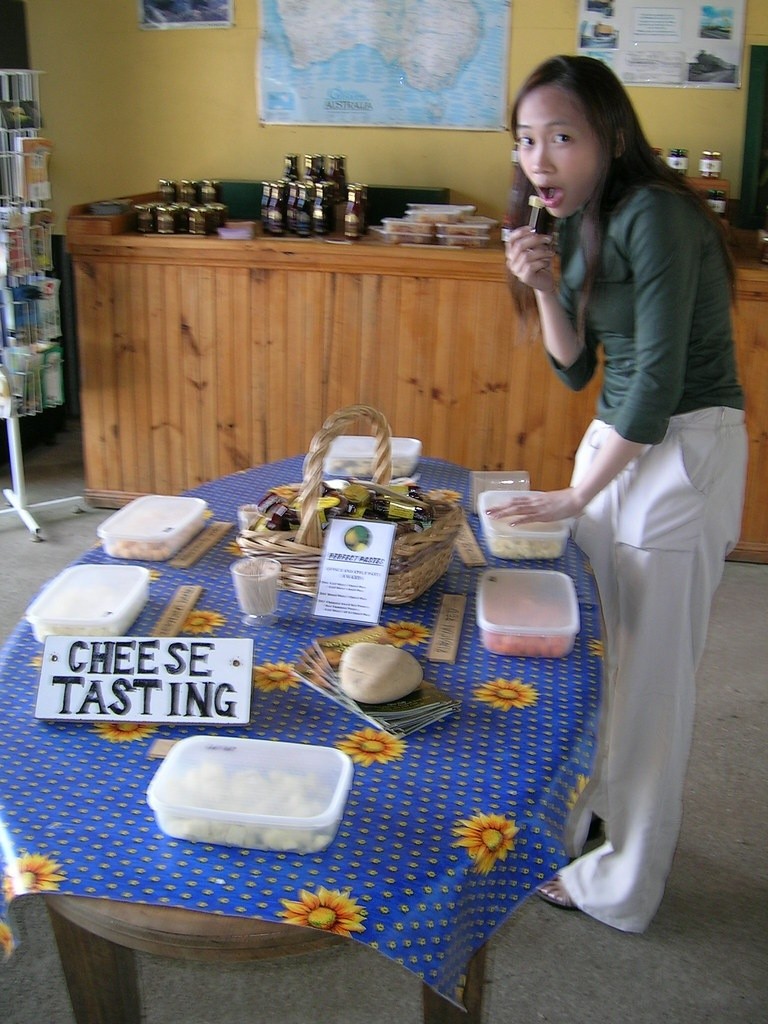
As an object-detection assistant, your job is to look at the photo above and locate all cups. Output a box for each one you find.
[230,557,282,627]
[237,506,258,530]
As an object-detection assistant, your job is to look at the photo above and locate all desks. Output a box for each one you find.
[66,178,768,564]
[0,455,606,1024]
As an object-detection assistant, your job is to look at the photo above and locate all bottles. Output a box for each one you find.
[134,178,226,235]
[260,152,369,241]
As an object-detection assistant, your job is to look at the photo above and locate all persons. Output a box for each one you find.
[484,56,749,935]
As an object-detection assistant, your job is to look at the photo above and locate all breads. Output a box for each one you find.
[339,642,423,704]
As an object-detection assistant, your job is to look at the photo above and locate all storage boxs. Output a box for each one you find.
[97,495,206,561]
[475,568,580,658]
[146,735,352,853]
[26,564,149,644]
[477,490,571,560]
[369,204,498,246]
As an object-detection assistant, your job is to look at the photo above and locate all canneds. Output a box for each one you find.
[705,189,726,219]
[134,177,226,236]
[651,148,722,179]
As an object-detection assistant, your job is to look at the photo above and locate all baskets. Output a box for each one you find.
[235,404,464,606]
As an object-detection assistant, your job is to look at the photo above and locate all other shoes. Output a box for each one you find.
[535,877,581,911]
[586,818,601,841]
